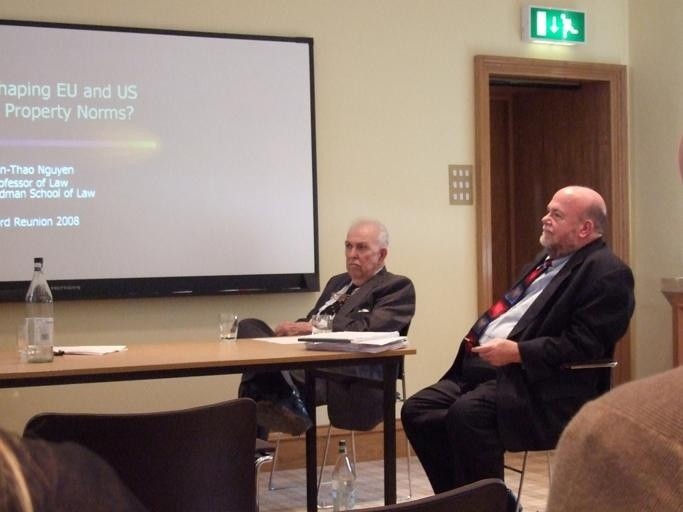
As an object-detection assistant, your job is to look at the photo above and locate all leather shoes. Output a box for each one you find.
[256,392,312,437]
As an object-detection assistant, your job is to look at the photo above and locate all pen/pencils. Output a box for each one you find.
[464,337,479,346]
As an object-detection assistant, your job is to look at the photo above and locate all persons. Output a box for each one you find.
[237,216,414,459]
[401,186,635,512]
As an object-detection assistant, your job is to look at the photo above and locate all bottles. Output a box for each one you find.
[25,257,53,363]
[332,440,355,511]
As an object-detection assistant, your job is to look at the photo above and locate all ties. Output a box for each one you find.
[317,283,359,329]
[464,258,554,354]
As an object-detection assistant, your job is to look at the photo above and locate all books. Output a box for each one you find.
[50,344,128,356]
[297,329,409,355]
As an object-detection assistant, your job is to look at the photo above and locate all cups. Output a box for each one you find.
[310,314,333,335]
[15,322,38,355]
[217,314,238,343]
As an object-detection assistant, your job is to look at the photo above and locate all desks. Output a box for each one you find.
[659,274,681,370]
[1,333,417,511]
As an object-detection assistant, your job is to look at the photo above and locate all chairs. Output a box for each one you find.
[268,316,415,511]
[19,397,257,509]
[505,339,615,511]
[332,476,524,512]
